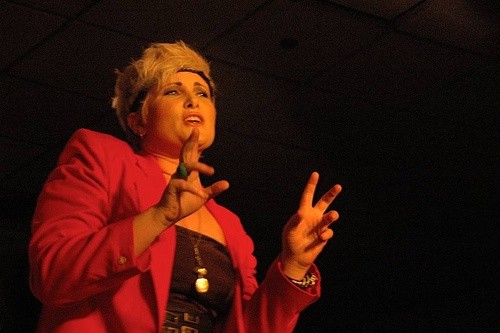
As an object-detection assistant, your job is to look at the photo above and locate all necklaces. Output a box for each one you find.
[161,162,212,294]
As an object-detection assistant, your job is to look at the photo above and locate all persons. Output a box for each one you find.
[28,39,344,332]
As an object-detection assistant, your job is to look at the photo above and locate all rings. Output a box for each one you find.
[176,160,191,178]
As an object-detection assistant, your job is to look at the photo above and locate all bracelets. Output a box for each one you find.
[283,270,319,290]
